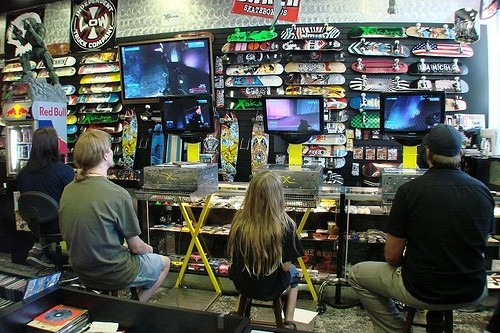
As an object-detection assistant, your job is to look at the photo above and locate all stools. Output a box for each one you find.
[236,285,291,333]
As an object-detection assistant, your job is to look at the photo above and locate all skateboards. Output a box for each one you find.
[201,23,473,186]
[0,51,200,181]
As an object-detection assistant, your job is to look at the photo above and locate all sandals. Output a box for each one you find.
[283,321,297,331]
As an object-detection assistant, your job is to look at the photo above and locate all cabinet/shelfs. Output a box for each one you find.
[135,182,500,310]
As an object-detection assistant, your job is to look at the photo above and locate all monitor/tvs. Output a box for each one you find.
[159,94,216,138]
[118,36,215,105]
[262,95,324,144]
[380,91,446,139]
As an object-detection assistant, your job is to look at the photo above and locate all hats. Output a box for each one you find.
[421,123,463,157]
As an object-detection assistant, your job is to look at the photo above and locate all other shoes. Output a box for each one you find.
[27,246,57,273]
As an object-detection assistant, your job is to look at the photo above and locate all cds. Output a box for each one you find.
[45,308,73,321]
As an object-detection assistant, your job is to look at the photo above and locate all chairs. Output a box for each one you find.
[18,191,65,276]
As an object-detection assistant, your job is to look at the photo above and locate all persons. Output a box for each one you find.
[57,128,171,302]
[13,17,59,85]
[16,126,74,271]
[347,124,496,333]
[227,171,302,330]
